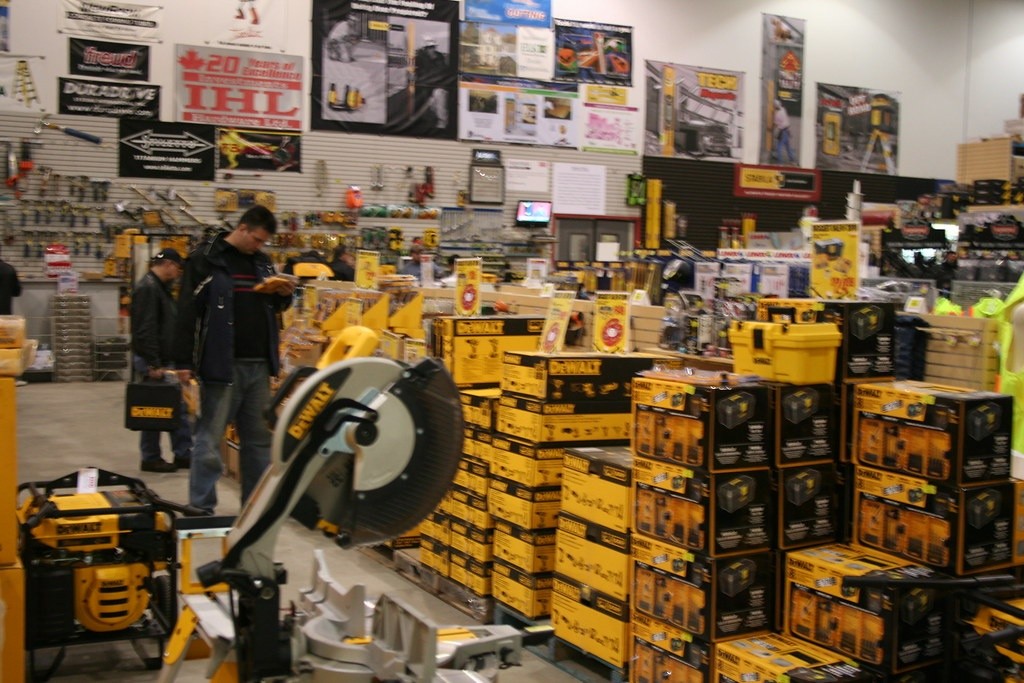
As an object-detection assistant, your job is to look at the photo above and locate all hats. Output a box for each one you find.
[421,34,438,49]
[150,248,186,266]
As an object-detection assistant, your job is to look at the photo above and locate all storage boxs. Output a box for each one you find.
[0,315,38,377]
[48,297,131,382]
[121,137,1024,683]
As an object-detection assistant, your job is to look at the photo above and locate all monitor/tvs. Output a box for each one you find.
[516,200,552,228]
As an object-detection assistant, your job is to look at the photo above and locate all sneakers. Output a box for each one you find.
[174,451,192,468]
[141,457,177,473]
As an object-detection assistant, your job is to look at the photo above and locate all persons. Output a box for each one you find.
[416,33,449,129]
[129,247,193,473]
[283,233,336,307]
[448,255,461,271]
[772,99,798,165]
[176,205,295,515]
[397,245,444,278]
[0,258,23,315]
[331,243,354,281]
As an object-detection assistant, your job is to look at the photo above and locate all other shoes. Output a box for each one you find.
[16,379,28,387]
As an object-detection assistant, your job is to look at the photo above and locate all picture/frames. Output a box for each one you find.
[470,165,504,204]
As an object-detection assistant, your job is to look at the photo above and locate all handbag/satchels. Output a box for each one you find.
[123,371,182,432]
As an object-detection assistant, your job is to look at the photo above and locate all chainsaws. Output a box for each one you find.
[192,344,563,683]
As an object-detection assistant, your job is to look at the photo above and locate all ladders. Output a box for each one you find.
[860,129,895,175]
[12,59,40,106]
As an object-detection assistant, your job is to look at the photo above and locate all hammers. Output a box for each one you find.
[16,166,116,260]
[114,180,212,227]
[34,111,104,143]
